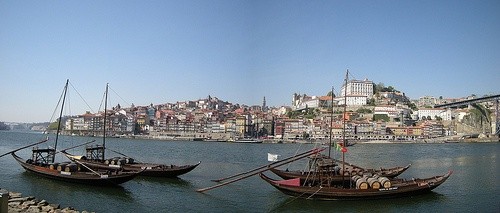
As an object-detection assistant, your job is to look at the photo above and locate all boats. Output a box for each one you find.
[321,137,358,148]
[191,135,263,144]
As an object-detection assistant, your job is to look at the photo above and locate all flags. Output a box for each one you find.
[337,145,347,152]
[334,142,343,147]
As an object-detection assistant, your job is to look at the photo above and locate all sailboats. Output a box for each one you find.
[265,86,415,187]
[259,69,454,202]
[11,78,148,188]
[60,82,202,178]
